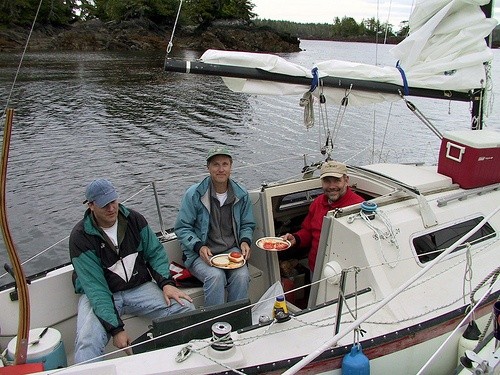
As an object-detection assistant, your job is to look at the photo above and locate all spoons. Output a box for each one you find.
[28,327,48,346]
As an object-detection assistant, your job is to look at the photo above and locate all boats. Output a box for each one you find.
[0,56,500,375]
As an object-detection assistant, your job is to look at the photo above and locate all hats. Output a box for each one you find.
[206,146,232,161]
[319,161,347,177]
[83,179,120,208]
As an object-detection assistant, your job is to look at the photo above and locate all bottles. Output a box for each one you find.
[273,295,287,317]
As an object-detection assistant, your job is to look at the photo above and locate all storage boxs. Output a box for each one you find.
[437,130,500,189]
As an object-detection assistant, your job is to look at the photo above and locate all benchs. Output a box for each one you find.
[103,261,263,360]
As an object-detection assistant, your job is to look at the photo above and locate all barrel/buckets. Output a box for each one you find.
[5,327,68,371]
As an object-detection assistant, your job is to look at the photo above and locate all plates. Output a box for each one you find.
[255,236,292,252]
[210,253,246,270]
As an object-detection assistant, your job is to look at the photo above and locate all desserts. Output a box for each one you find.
[228,252,243,263]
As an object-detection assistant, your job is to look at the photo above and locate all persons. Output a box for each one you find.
[280,160,367,280]
[69,177,196,364]
[173,146,257,307]
